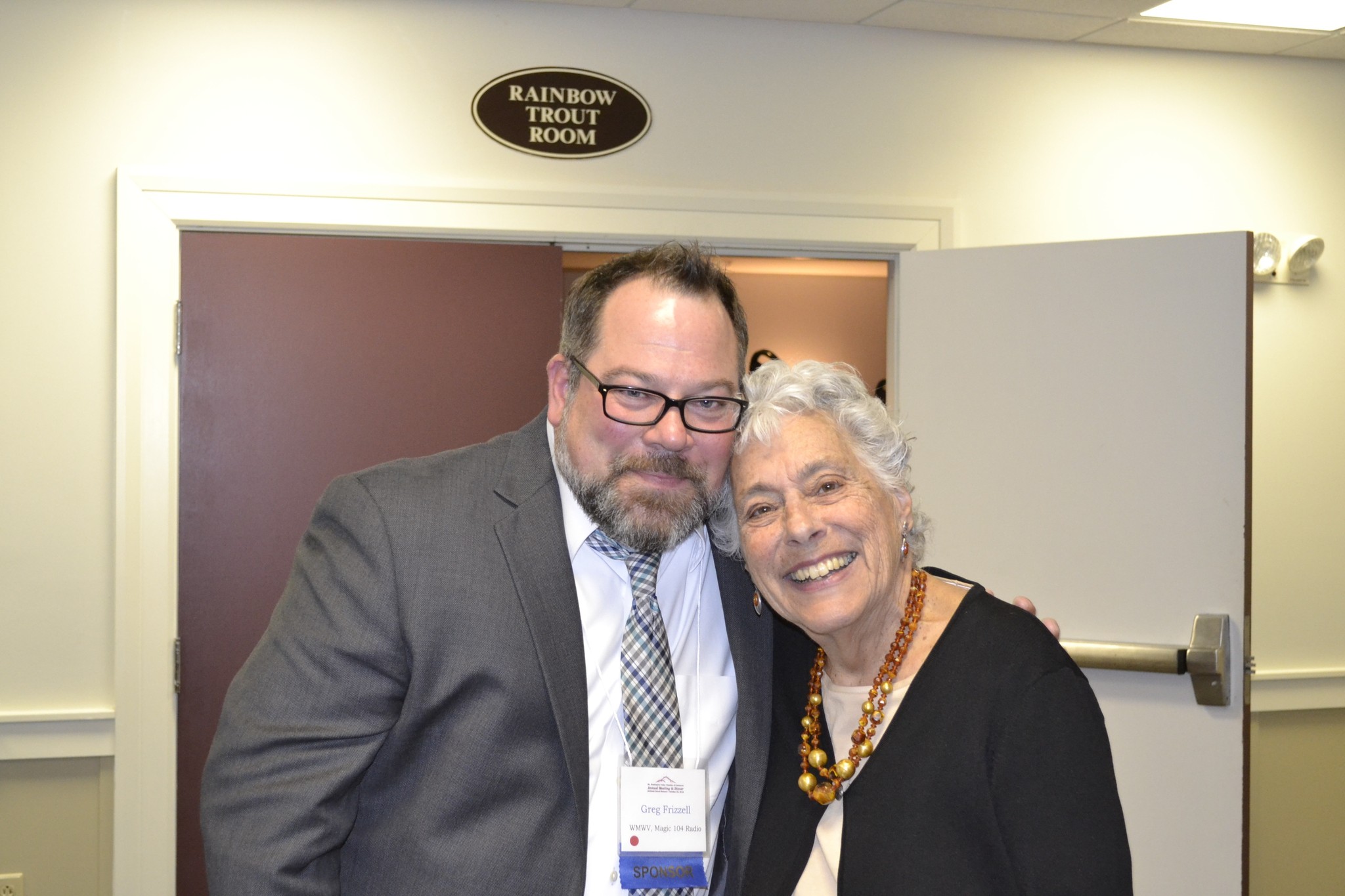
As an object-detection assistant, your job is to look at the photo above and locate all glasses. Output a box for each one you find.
[569,354,750,434]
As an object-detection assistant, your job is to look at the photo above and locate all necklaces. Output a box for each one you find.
[793,565,929,806]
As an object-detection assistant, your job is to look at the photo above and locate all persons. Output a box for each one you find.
[200,241,781,894]
[706,356,1139,896]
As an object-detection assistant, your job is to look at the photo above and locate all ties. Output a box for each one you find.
[585,529,696,896]
